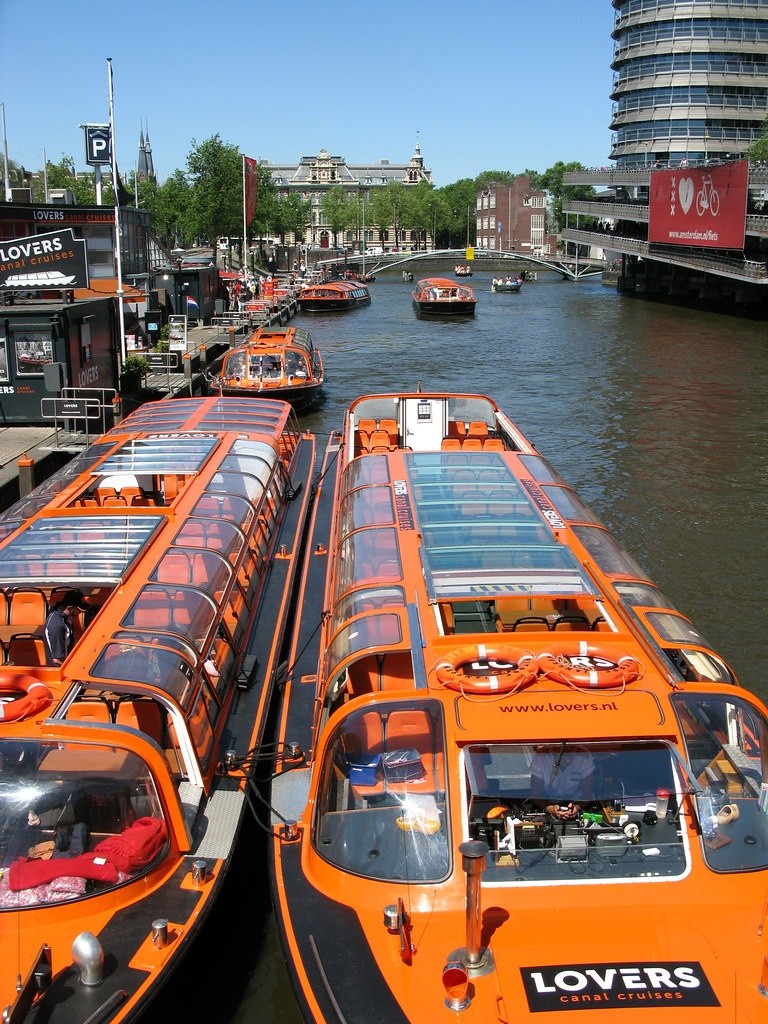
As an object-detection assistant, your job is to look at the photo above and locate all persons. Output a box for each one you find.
[42,589,90,666]
[574,155,768,264]
[290,263,522,299]
[148,621,183,687]
[16,347,38,360]
[250,354,302,372]
[529,744,593,820]
[226,275,272,311]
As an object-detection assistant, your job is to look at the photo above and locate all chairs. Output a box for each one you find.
[74,777,135,834]
[343,420,614,809]
[0,434,296,754]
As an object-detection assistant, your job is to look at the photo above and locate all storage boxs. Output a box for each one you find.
[345,754,381,786]
[381,748,423,782]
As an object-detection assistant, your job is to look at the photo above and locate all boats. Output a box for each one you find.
[296,280,372,314]
[454,273,473,277]
[209,327,324,412]
[412,278,479,316]
[494,284,521,292]
[0,384,317,1024]
[271,379,768,1024]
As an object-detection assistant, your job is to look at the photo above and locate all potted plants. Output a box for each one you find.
[121,355,150,392]
[153,324,171,354]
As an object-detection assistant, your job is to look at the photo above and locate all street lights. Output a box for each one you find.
[269,244,277,289]
[172,247,186,313]
[249,245,257,276]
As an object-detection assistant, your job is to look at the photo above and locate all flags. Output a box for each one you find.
[187,296,200,308]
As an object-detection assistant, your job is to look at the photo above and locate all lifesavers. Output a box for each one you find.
[436,643,539,695]
[537,640,639,688]
[0,671,53,722]
[253,343,274,348]
